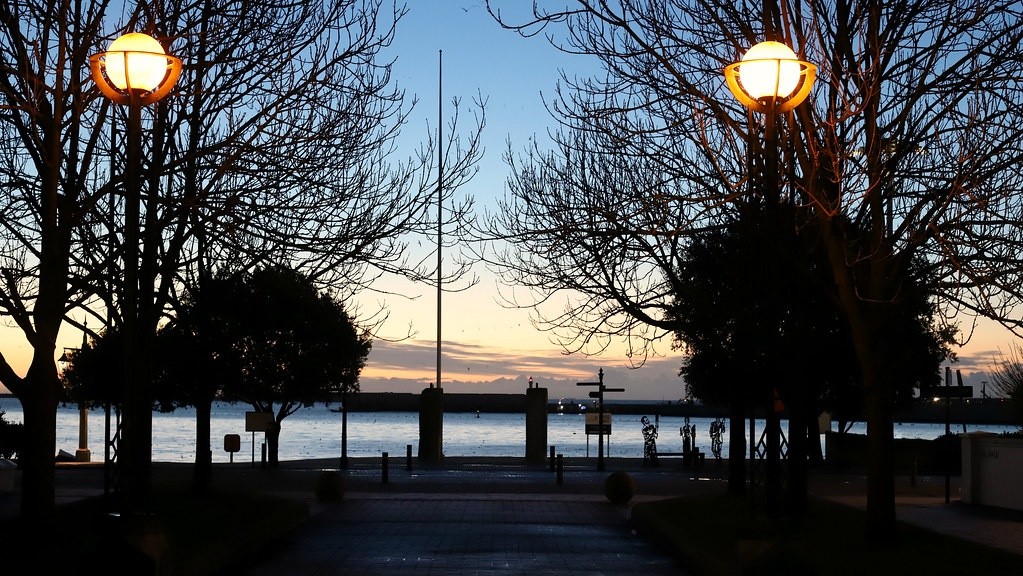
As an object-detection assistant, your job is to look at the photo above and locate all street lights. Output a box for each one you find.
[709,38,819,535]
[88,31,184,536]
